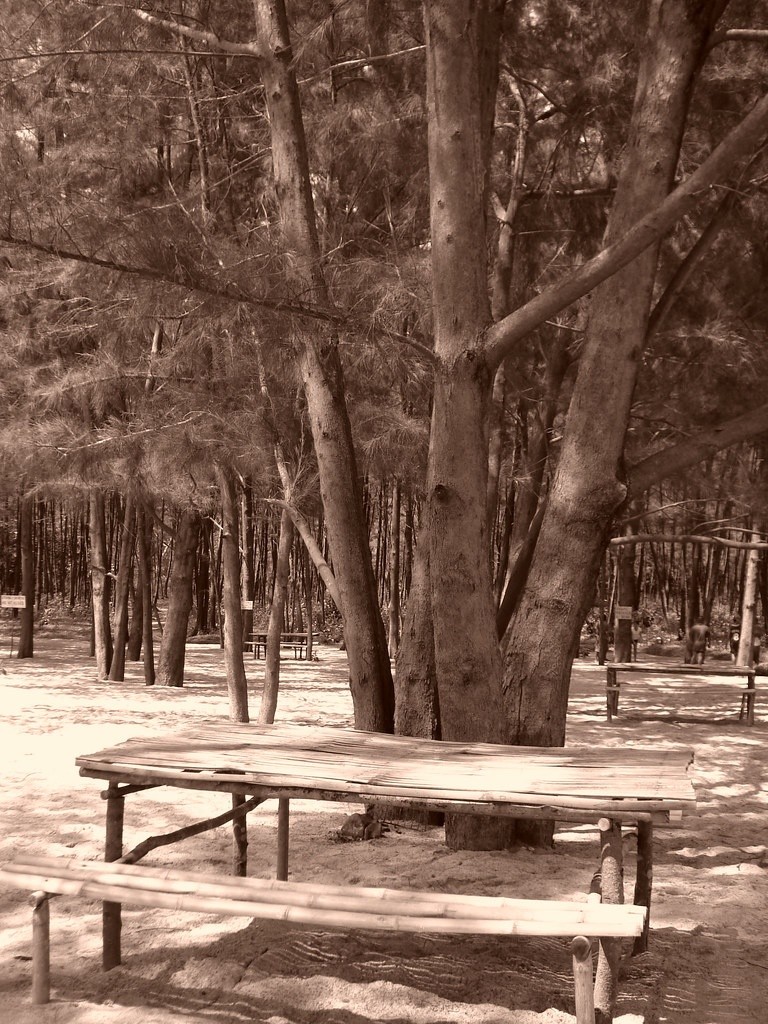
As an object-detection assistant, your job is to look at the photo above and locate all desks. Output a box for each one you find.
[248,633,320,659]
[602,665,757,716]
[74,720,699,984]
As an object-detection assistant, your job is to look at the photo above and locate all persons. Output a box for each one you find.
[753,618,763,665]
[594,614,611,662]
[688,616,711,665]
[727,616,741,666]
[631,622,642,644]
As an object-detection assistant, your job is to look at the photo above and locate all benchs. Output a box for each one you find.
[244,641,318,660]
[0,853,648,1024]
[604,683,756,727]
